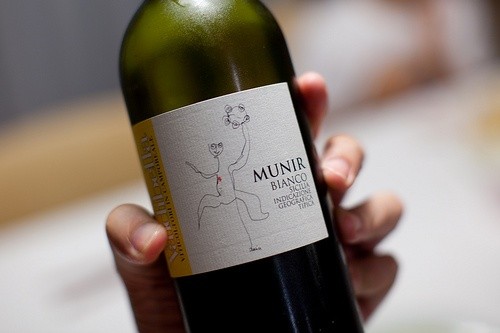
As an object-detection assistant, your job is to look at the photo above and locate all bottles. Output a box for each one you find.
[118,0,364,332]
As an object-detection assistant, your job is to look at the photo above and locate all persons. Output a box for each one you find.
[104,68,404,332]
